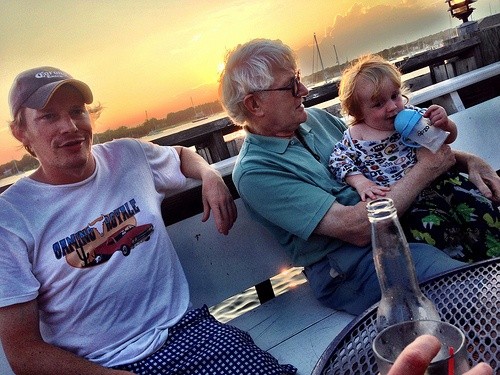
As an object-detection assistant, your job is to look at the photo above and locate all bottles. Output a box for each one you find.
[366,198,441,358]
[393,108,450,155]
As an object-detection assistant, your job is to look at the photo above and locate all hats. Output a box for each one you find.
[8,67,93,117]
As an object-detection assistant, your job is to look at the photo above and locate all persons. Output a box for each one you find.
[0,66,302,375]
[387,335,494,375]
[329,55,500,265]
[218,38,500,323]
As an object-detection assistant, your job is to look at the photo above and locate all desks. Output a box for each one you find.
[310,256,499,374]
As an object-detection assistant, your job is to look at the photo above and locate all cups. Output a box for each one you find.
[371,319,470,375]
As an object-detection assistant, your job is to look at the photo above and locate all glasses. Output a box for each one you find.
[248,68,301,98]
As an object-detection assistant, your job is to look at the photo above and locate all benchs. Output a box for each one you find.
[0,60,500,374]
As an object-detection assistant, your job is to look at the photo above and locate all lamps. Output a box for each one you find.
[445,0,476,23]
